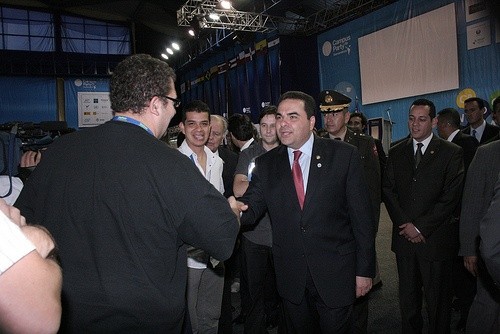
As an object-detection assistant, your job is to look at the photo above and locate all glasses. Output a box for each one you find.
[150,95,182,108]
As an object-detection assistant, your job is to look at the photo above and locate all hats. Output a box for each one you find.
[319,90,352,113]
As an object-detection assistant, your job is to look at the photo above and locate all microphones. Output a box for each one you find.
[23,120,68,130]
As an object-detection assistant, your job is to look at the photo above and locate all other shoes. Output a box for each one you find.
[371,280,383,290]
[266,312,279,329]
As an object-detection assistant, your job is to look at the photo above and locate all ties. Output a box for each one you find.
[291,150,306,211]
[472,130,477,140]
[335,138,341,140]
[415,143,424,168]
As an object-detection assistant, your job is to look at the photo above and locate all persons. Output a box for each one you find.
[0,55,239,334]
[384,96,500,334]
[229,89,386,334]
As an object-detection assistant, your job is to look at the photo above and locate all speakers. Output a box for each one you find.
[368,117,390,155]
